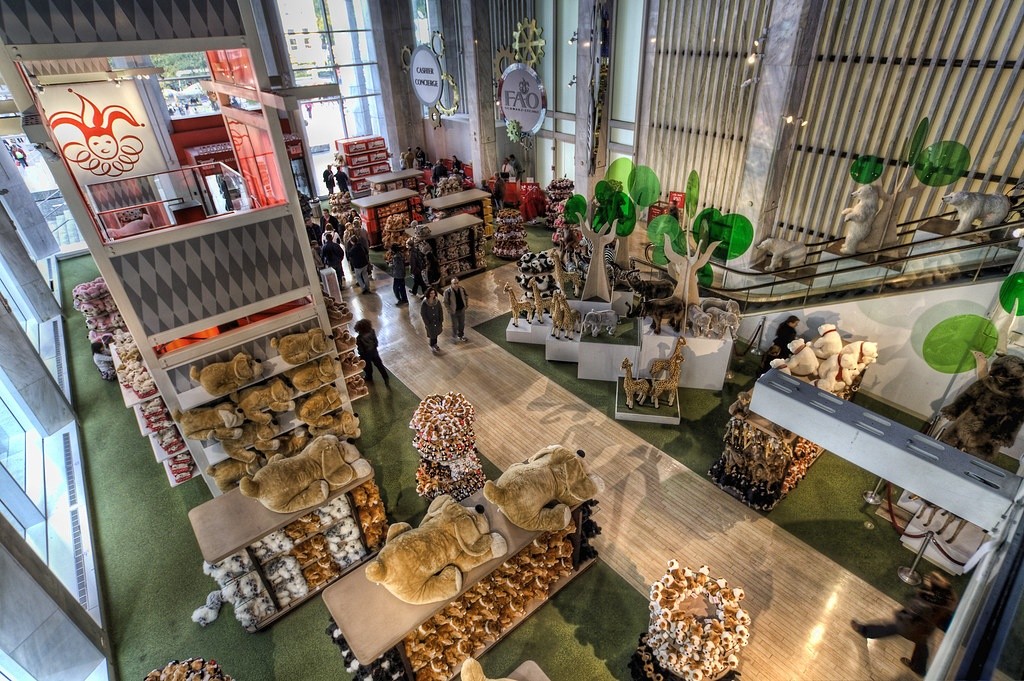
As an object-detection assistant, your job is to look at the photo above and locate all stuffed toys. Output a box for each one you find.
[371,175,742,328]
[942,191,1011,237]
[73,277,127,343]
[319,282,353,327]
[887,237,963,288]
[106,208,152,238]
[756,237,807,272]
[110,328,756,681]
[839,183,879,255]
[940,352,1024,463]
[757,323,880,512]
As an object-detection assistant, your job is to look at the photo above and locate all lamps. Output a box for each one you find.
[800,116,808,126]
[748,52,756,63]
[568,37,576,45]
[568,80,573,87]
[754,36,765,45]
[782,115,793,123]
[34,142,61,164]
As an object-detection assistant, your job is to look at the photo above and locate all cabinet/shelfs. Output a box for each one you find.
[713,333,867,512]
[77,291,370,487]
[187,452,383,634]
[321,458,600,681]
[335,144,493,285]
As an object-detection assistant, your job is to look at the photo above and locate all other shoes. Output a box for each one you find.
[451,336,469,345]
[900,657,925,677]
[420,295,427,300]
[384,377,389,385]
[851,620,867,638]
[428,343,439,353]
[363,377,372,381]
[395,300,409,306]
[339,280,372,294]
[409,290,416,296]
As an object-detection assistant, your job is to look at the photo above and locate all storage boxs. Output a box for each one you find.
[335,133,392,191]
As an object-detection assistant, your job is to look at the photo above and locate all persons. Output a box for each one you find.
[493,154,524,175]
[90,336,118,382]
[430,154,463,176]
[772,316,800,358]
[399,146,426,171]
[160,78,239,118]
[297,162,374,295]
[2,137,29,169]
[354,319,390,386]
[850,570,960,677]
[305,94,348,119]
[216,173,243,211]
[915,497,969,544]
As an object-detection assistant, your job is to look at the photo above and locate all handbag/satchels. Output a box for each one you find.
[768,345,782,357]
